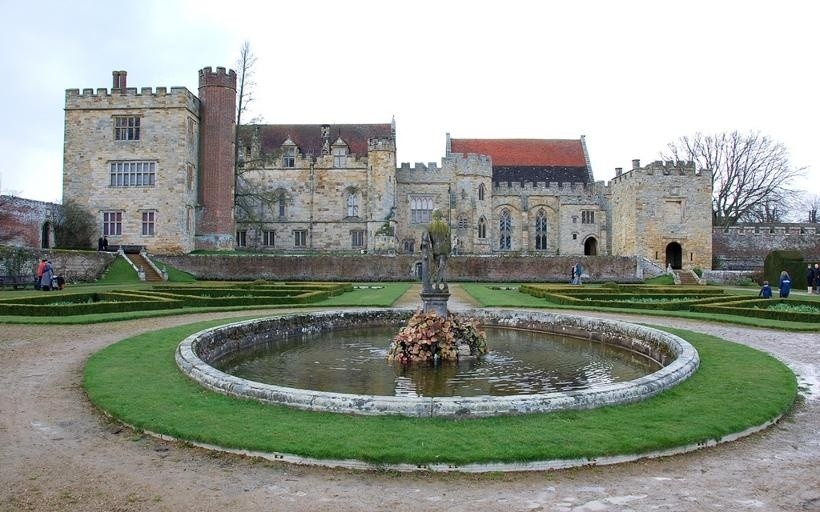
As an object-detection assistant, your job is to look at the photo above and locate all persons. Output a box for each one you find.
[807,263,815,288]
[573,262,582,285]
[758,280,773,299]
[778,270,792,298]
[103,236,108,251]
[36,258,66,292]
[420,208,454,293]
[98,234,104,251]
[813,263,820,293]
[571,264,575,285]
